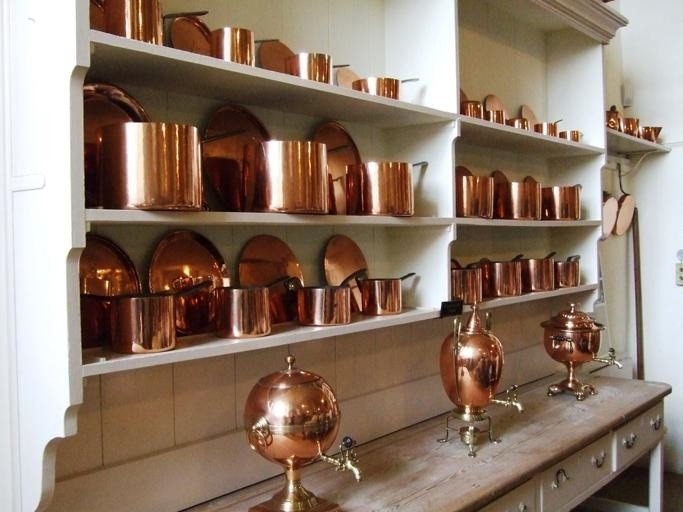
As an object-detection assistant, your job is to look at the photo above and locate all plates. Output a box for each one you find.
[521,105,539,132]
[202,103,271,210]
[237,234,305,325]
[170,16,213,56]
[84,82,155,212]
[148,230,232,336]
[259,41,293,74]
[336,69,359,90]
[80,231,144,346]
[460,89,468,114]
[90,0,105,31]
[313,120,363,216]
[322,234,369,313]
[484,94,508,126]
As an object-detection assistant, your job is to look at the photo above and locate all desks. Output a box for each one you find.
[184,370,672,512]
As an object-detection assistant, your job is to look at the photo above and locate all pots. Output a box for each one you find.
[303,268,370,328]
[451,174,582,306]
[213,275,292,337]
[98,120,250,214]
[534,117,563,141]
[208,28,257,68]
[104,1,208,46]
[254,138,330,216]
[109,277,213,354]
[366,271,416,317]
[352,75,420,103]
[283,52,350,90]
[344,159,428,216]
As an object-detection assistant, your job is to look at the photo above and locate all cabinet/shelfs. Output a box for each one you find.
[81,0,672,379]
